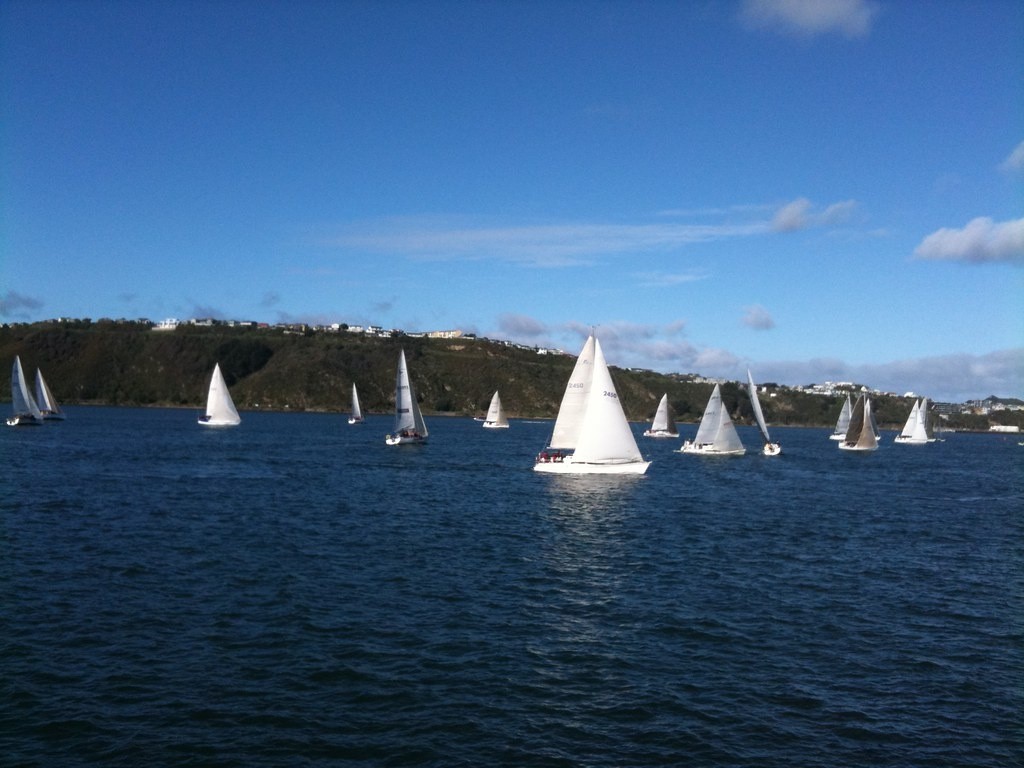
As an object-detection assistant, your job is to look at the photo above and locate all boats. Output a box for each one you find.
[473,416,486,421]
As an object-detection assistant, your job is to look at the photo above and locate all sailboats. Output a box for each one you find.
[4,355,68,426]
[644,393,679,438]
[680,383,746,457]
[198,363,242,429]
[482,390,510,428]
[385,348,428,446]
[746,369,781,456]
[347,383,365,424]
[829,392,881,452]
[894,397,946,445]
[531,328,653,475]
